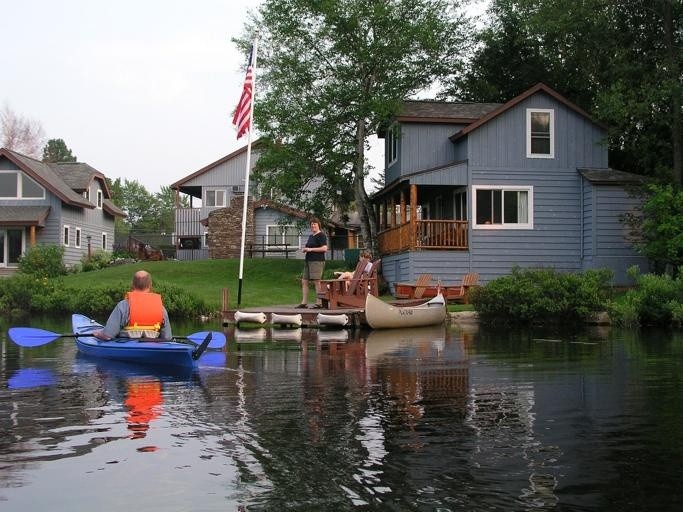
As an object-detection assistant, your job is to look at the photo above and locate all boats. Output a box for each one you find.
[71,313,212,365]
[365,292,447,328]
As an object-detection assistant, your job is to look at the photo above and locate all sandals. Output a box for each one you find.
[309,303,323,309]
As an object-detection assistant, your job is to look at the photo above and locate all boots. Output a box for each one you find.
[294,303,308,309]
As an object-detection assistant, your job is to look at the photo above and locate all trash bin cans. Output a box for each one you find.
[345,249,359,269]
[161,249,168,261]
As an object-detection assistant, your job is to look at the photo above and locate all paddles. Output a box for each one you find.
[8,328,226,348]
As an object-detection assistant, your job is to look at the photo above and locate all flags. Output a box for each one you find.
[232,45,253,140]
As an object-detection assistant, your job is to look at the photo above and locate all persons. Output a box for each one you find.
[93,271,172,341]
[327,252,373,291]
[294,218,327,309]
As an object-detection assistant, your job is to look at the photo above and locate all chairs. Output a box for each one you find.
[316,259,382,310]
[392,271,481,307]
